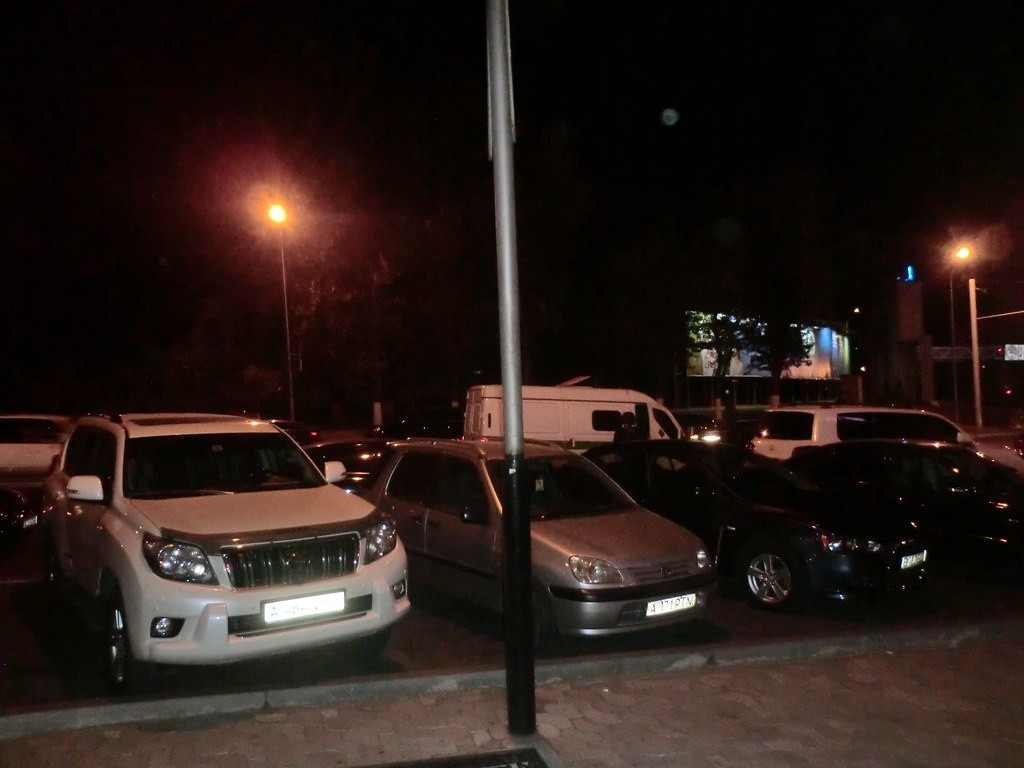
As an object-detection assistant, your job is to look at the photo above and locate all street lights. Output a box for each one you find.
[263,200,300,421]
[950,240,988,430]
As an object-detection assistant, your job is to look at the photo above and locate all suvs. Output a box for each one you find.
[40,413,410,701]
[754,402,1023,486]
[0,415,78,489]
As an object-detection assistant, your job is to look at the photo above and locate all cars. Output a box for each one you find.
[0,485,49,590]
[368,415,466,443]
[696,418,757,461]
[577,438,941,625]
[785,442,1024,598]
[301,436,404,499]
[378,438,717,649]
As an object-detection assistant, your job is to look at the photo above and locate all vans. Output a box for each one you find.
[462,375,685,448]
